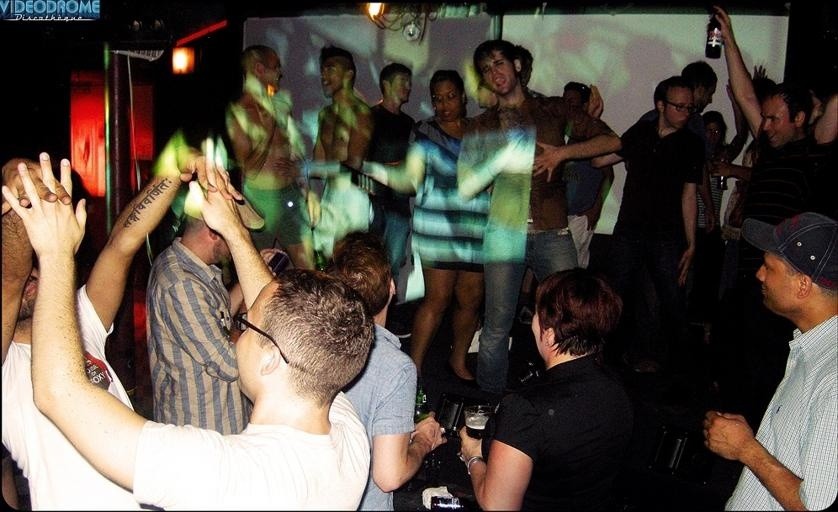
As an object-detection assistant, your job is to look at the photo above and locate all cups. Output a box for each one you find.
[462,403,501,440]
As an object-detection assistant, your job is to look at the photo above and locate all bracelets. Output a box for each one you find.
[465,455,483,474]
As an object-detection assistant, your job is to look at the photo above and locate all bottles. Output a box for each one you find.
[705,10,725,60]
[415,382,429,423]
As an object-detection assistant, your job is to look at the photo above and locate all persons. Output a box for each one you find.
[325,228,452,512]
[220,2,838,392]
[0,150,245,511]
[697,203,838,511]
[145,173,277,440]
[457,266,639,511]
[4,155,378,511]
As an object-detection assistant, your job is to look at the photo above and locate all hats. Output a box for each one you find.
[737,209,838,292]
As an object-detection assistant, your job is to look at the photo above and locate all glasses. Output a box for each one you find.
[662,99,697,114]
[228,310,297,366]
